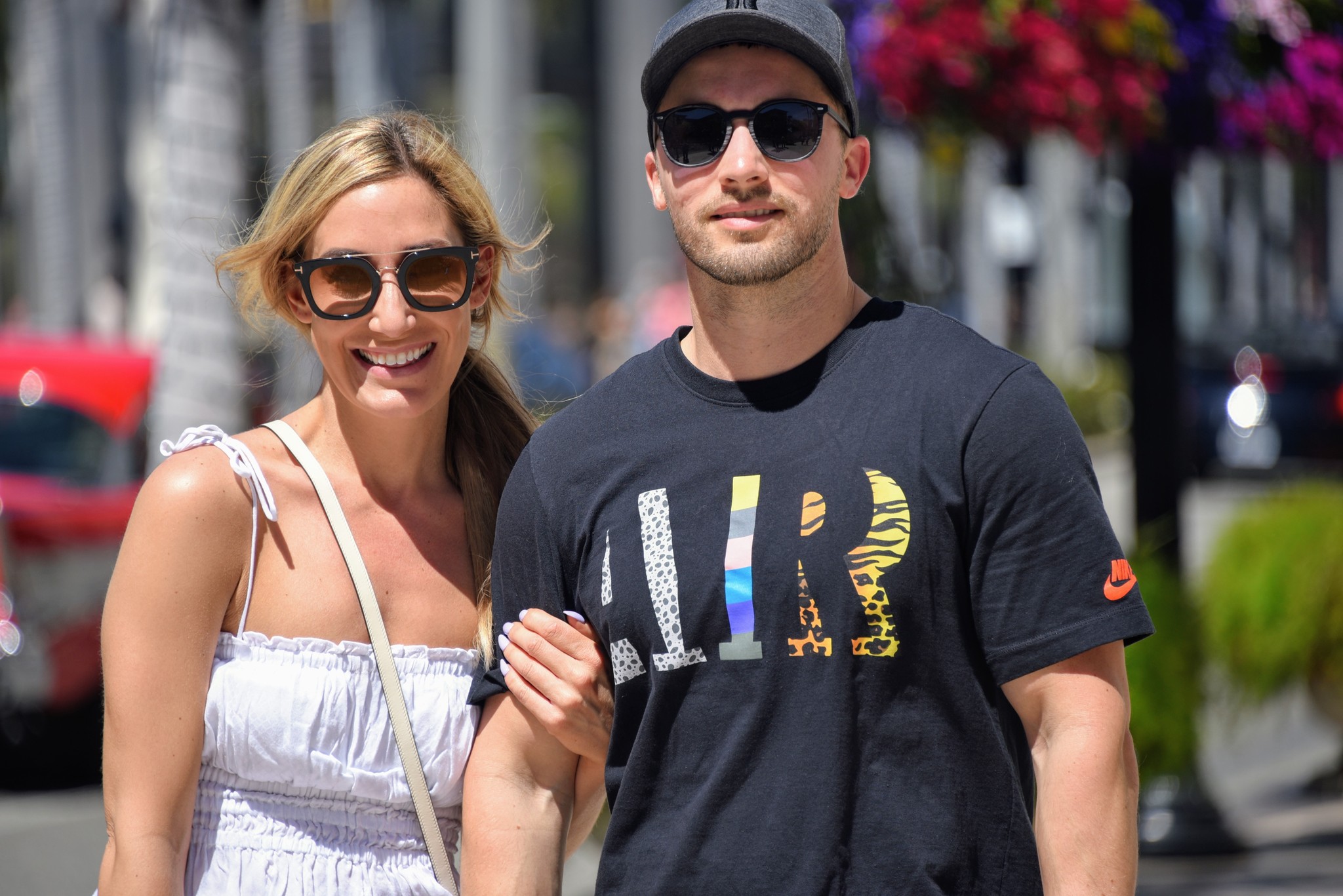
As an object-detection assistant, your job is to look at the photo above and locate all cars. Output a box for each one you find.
[1187,311,1343,489]
[0,325,168,797]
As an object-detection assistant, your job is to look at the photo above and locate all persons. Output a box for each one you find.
[458,0,1155,896]
[92,103,613,896]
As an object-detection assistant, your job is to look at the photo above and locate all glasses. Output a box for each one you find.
[290,245,481,320]
[654,96,857,168]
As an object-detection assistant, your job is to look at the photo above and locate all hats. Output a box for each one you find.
[642,1,858,134]
